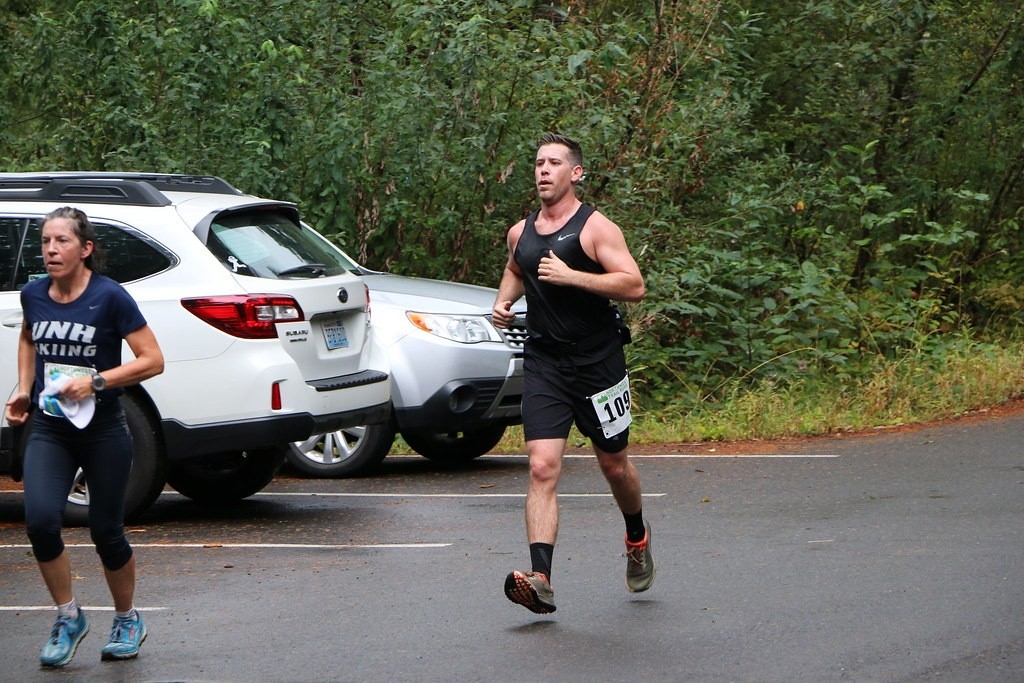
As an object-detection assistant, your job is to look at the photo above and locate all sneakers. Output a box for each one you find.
[504,570,557,614]
[101,609,147,659]
[39,607,88,666]
[625,519,655,592]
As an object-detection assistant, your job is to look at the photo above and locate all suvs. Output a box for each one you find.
[0,171,390,529]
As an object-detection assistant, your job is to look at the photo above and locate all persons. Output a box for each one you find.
[490,133,654,614]
[5,206,165,668]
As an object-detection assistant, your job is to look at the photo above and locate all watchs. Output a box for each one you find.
[90,371,107,395]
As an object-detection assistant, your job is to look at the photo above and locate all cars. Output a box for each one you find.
[282,221,528,478]
